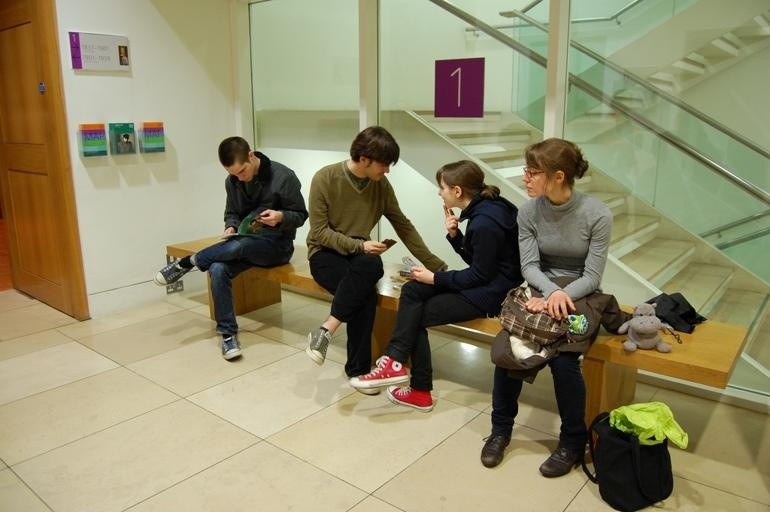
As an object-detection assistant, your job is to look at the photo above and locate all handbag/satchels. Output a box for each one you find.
[498,285,572,345]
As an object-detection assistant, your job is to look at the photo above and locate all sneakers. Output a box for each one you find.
[221,332,243,361]
[538,441,587,480]
[348,355,409,388]
[479,427,514,469]
[385,382,435,413]
[345,375,382,396]
[238,354,241,356]
[303,326,333,366]
[397,369,399,370]
[152,255,193,288]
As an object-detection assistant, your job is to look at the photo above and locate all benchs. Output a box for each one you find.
[162,234,751,440]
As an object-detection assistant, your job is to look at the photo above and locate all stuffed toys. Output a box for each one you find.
[617,303,674,353]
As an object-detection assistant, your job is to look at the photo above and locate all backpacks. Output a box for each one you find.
[580,408,677,512]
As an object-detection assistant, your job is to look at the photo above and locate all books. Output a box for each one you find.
[220,207,283,238]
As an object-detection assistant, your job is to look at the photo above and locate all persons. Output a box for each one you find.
[116,133,132,154]
[305,126,448,396]
[350,161,524,412]
[152,136,309,361]
[481,139,613,478]
[120,47,128,65]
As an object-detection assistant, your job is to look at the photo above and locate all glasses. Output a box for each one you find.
[521,165,544,178]
